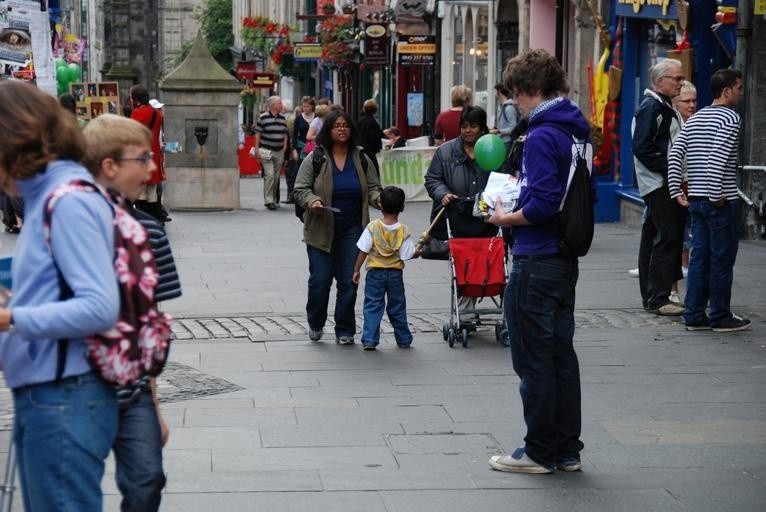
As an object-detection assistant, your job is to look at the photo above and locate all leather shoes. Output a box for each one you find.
[268,203,276,210]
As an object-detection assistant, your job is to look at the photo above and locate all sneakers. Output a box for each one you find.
[628,264,752,332]
[488,447,557,474]
[308,329,410,349]
[560,459,581,472]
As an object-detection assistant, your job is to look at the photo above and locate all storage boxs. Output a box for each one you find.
[591,175,620,223]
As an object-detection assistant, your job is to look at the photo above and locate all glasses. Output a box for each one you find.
[662,74,686,82]
[117,152,154,164]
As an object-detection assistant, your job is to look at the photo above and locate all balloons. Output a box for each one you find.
[473,134,506,171]
[55,58,80,98]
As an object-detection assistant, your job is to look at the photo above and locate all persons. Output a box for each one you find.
[355,99,406,181]
[60,95,86,127]
[425,105,513,336]
[238,122,245,150]
[129,85,172,221]
[352,186,421,349]
[0,189,21,234]
[487,83,519,174]
[435,84,472,145]
[82,114,181,511]
[631,58,753,332]
[293,111,383,342]
[484,48,594,473]
[0,80,117,512]
[256,96,342,209]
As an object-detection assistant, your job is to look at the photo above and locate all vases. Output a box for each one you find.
[237,136,262,176]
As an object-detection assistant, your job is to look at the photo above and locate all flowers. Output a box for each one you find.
[240,85,261,135]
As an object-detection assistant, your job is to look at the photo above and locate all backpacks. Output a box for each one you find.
[543,122,594,258]
[43,180,170,402]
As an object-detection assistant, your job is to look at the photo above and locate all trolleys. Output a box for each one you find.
[440,195,518,347]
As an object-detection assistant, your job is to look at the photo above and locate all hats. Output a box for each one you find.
[148,99,164,110]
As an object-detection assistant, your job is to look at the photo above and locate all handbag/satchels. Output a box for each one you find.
[422,239,451,259]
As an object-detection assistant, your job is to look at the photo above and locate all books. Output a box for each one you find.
[163,142,179,153]
